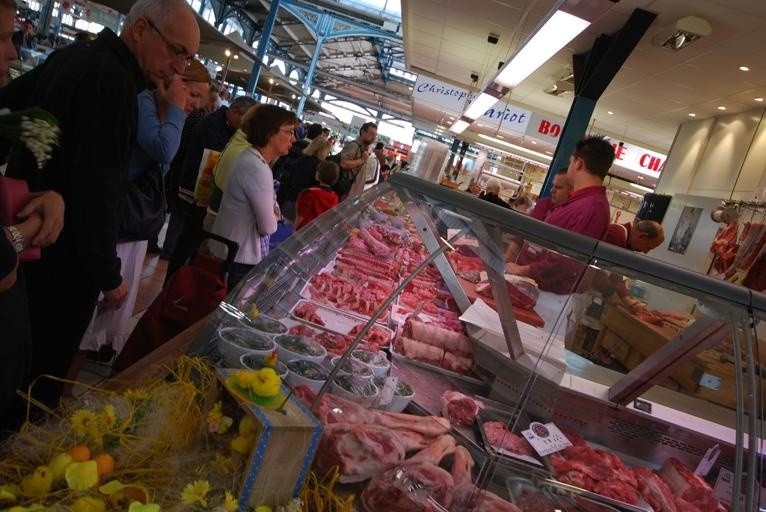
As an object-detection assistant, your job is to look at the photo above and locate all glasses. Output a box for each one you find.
[278,127,295,137]
[144,17,195,62]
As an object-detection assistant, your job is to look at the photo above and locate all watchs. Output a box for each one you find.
[5,224,25,254]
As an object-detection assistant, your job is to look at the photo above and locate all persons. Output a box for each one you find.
[328,135,335,153]
[202,103,287,241]
[504,132,615,295]
[294,160,340,231]
[364,142,385,192]
[77,60,210,377]
[13,19,34,49]
[302,123,328,161]
[208,104,296,294]
[592,219,666,313]
[161,95,257,284]
[295,118,309,139]
[385,151,410,172]
[321,128,330,138]
[1,0,66,293]
[340,122,378,201]
[181,87,220,136]
[222,93,231,106]
[464,180,481,196]
[0,0,201,434]
[505,167,572,263]
[380,165,391,183]
[483,180,510,208]
[513,195,532,213]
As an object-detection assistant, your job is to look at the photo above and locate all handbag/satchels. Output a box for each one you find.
[119,103,169,248]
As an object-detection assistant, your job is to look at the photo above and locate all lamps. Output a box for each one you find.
[461,87,505,124]
[488,0,620,96]
[449,116,471,135]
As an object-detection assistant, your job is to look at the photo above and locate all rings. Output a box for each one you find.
[181,83,189,89]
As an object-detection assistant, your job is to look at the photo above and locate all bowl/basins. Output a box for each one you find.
[218,327,276,367]
[239,353,289,381]
[287,358,329,393]
[330,355,375,379]
[238,313,289,338]
[371,375,416,412]
[273,334,327,364]
[351,350,390,376]
[331,374,378,408]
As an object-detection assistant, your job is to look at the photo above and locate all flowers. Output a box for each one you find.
[0,382,252,512]
